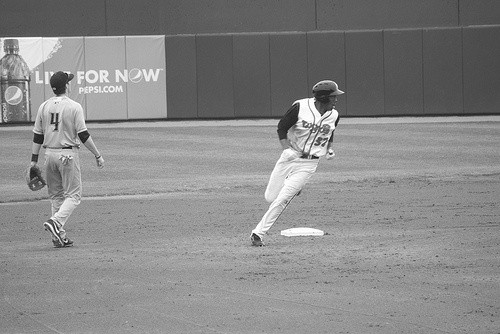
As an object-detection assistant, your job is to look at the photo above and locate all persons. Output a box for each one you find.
[250,79,345,247]
[29,72,104,248]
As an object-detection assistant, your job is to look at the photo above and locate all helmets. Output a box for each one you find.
[312,80,344,103]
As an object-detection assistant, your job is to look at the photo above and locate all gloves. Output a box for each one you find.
[283,148,299,161]
[326,147,336,161]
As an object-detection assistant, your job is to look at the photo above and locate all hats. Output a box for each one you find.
[50,71,74,90]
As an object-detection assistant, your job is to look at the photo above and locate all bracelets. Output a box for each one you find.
[95,155,101,159]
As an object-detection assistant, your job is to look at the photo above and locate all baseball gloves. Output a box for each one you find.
[25,165,46,192]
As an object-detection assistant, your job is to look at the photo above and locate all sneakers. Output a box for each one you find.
[251,233,266,247]
[43,218,65,247]
[52,237,74,247]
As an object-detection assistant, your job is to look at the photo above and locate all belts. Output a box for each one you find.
[62,146,72,149]
[300,154,319,159]
